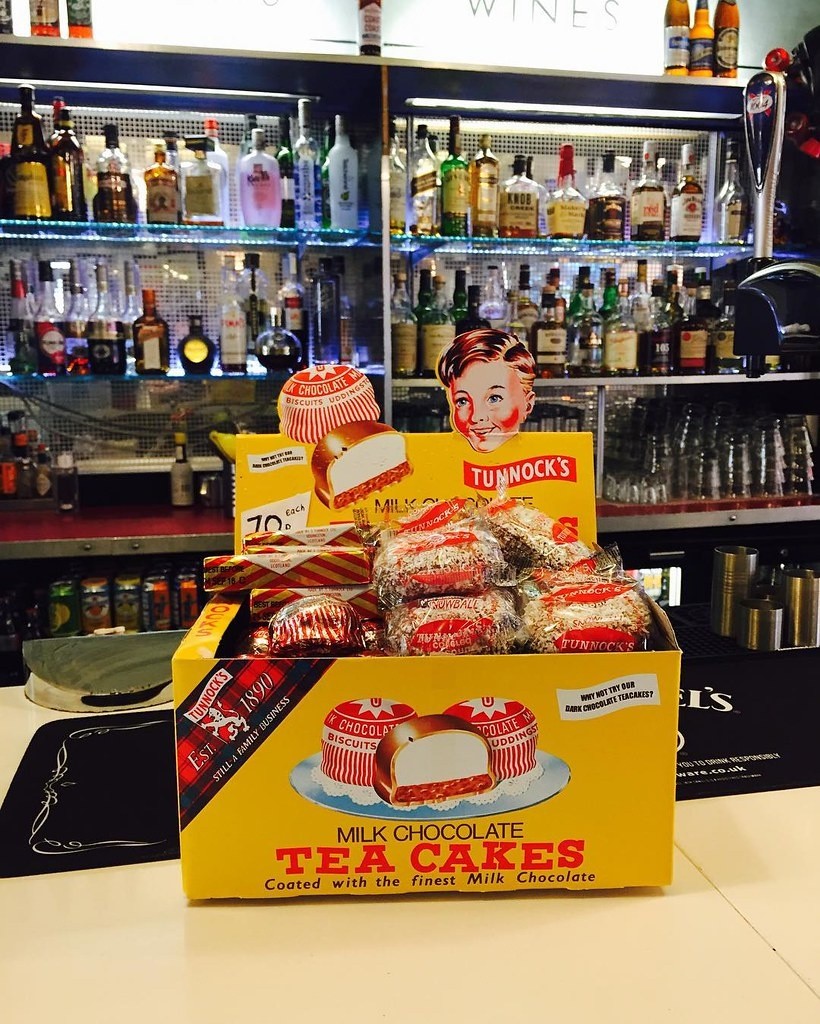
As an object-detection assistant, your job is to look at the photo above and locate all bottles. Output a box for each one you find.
[92,124,139,223]
[254,254,308,367]
[8,84,90,221]
[357,0,382,57]
[389,114,751,244]
[663,0,740,78]
[0,0,94,39]
[7,257,170,376]
[177,313,217,376]
[55,455,78,513]
[143,119,229,224]
[392,402,582,432]
[302,256,357,366]
[169,432,193,509]
[220,254,269,371]
[391,255,747,377]
[0,429,52,499]
[239,111,372,230]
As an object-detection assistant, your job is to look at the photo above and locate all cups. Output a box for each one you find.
[199,474,223,508]
[604,404,816,502]
[711,546,820,651]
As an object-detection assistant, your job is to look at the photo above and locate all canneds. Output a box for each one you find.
[47,560,201,638]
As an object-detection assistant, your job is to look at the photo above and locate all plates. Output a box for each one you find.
[286,756,571,824]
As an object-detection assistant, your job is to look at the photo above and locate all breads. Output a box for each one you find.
[371,502,654,655]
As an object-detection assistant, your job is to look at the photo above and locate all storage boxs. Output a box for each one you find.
[173,432,683,900]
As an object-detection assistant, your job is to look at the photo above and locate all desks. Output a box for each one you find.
[1,650,820,1023]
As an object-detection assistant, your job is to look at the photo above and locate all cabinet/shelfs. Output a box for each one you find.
[0,34,820,690]
